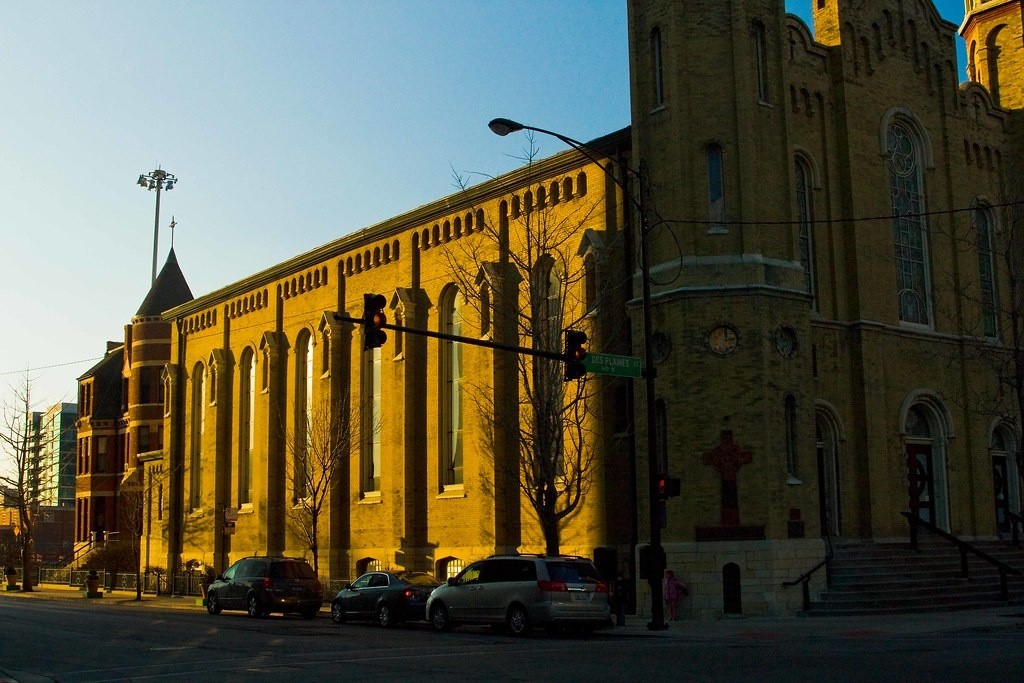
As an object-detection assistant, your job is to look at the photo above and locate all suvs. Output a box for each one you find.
[423,553,613,637]
[204,555,325,619]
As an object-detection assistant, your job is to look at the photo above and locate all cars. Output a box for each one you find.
[330,571,443,630]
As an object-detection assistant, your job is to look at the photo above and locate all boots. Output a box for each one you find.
[673,608,678,620]
[668,609,672,620]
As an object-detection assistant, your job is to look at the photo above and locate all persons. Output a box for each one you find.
[663,570,688,621]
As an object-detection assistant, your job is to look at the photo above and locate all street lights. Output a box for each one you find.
[136,164,178,292]
[488,117,671,633]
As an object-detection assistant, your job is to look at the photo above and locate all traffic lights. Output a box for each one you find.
[564,329,586,383]
[362,292,391,353]
[657,477,682,499]
